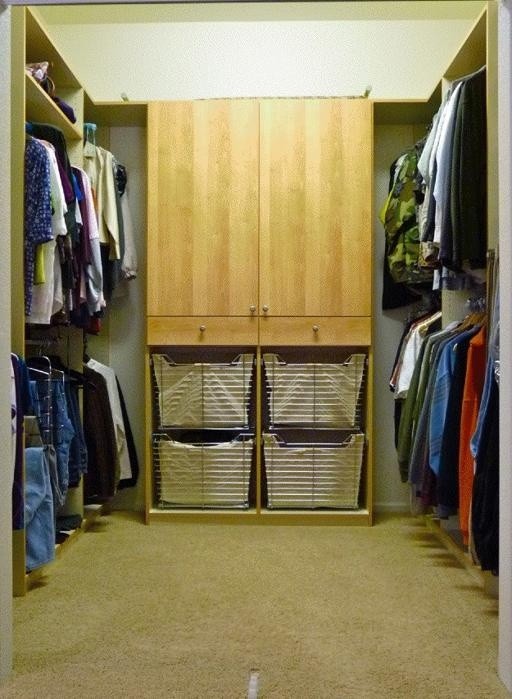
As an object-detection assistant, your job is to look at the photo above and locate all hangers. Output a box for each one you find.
[448,297,488,335]
[11,339,100,390]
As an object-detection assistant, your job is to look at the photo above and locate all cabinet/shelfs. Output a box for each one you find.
[145,96,373,526]
[425,4,499,598]
[11,6,111,595]
